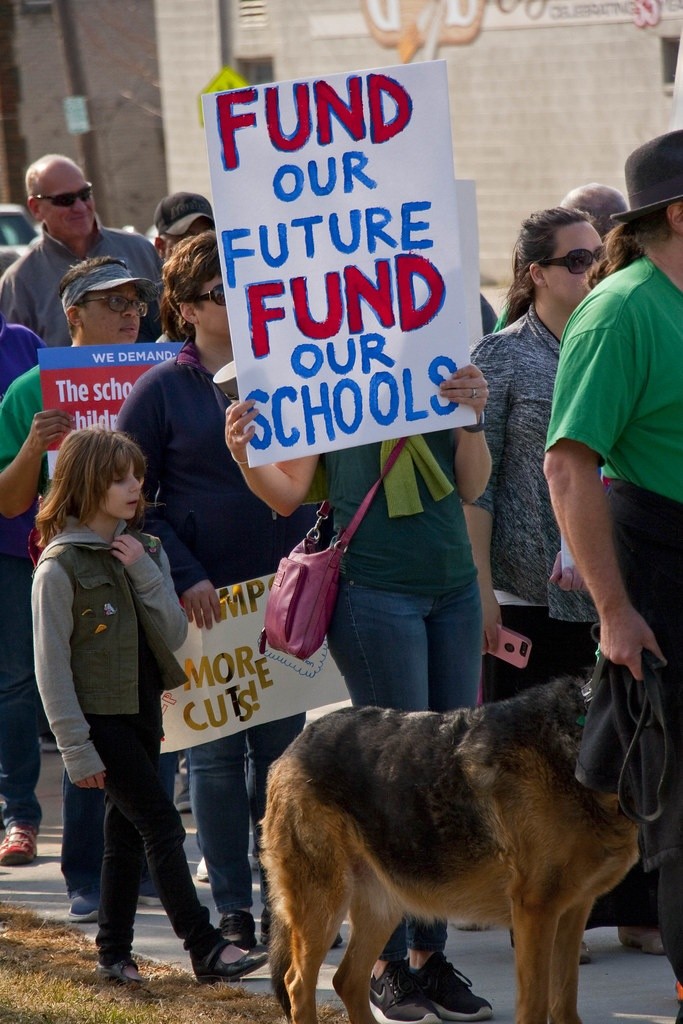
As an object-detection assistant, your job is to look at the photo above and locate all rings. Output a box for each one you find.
[232,423,242,433]
[472,389,477,398]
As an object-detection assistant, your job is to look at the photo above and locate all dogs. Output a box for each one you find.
[260,678,640,1024]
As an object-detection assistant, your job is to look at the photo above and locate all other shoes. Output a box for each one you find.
[175,775,192,812]
[617,925,667,955]
[95,959,146,988]
[216,910,257,947]
[192,940,268,982]
[260,909,343,948]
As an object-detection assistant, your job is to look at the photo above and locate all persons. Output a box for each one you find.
[32,425,266,982]
[226,363,493,1023]
[1,129,683,1024]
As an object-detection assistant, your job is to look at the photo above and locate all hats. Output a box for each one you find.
[153,192,215,236]
[60,264,161,316]
[609,129,683,221]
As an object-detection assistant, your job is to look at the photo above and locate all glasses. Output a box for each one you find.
[538,245,608,274]
[76,295,148,316]
[34,181,93,207]
[183,284,226,306]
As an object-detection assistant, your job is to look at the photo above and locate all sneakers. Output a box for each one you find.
[406,952,494,1020]
[68,885,101,922]
[0,823,38,865]
[138,880,163,906]
[369,959,443,1024]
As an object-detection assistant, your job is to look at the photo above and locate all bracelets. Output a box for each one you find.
[463,413,484,432]
[231,456,248,464]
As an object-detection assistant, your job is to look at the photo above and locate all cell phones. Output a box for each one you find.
[488,624,533,669]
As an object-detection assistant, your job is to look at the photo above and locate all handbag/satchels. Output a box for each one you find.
[263,539,344,659]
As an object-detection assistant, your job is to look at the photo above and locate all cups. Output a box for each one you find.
[213,361,238,402]
